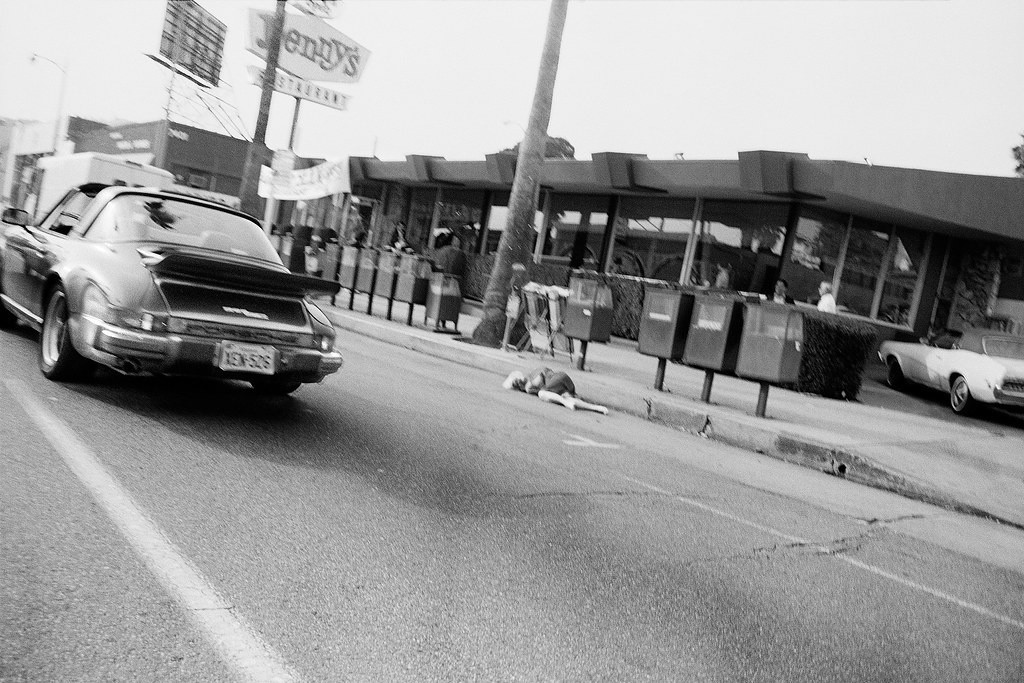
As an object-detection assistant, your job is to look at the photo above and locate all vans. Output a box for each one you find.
[20,151,176,224]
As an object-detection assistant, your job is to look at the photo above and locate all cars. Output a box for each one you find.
[0,179,345,397]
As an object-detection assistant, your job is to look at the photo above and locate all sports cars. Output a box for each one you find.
[875,323,1024,418]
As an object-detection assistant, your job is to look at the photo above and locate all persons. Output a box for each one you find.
[817,280,837,315]
[6,209,19,223]
[439,236,467,281]
[390,221,408,253]
[765,279,796,305]
[881,302,911,326]
[503,366,608,415]
[714,258,729,289]
